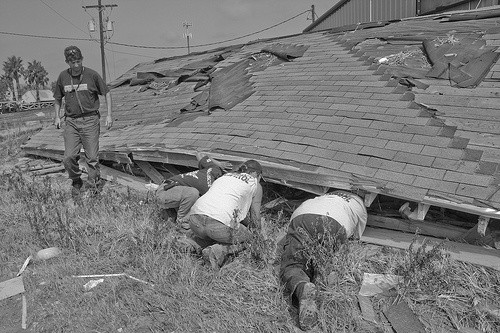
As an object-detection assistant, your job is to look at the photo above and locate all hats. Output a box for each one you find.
[63,46,83,60]
[239,160,266,183]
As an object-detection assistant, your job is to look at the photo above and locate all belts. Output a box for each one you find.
[72,110,99,117]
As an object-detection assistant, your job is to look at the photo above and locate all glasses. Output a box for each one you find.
[64,48,81,55]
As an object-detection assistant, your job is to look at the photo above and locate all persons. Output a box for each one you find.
[280,190,368,332]
[186,160,263,272]
[54,45,113,196]
[156,155,224,231]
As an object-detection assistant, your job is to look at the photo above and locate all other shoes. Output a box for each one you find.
[71,178,84,196]
[175,218,192,231]
[200,247,220,273]
[295,281,318,331]
